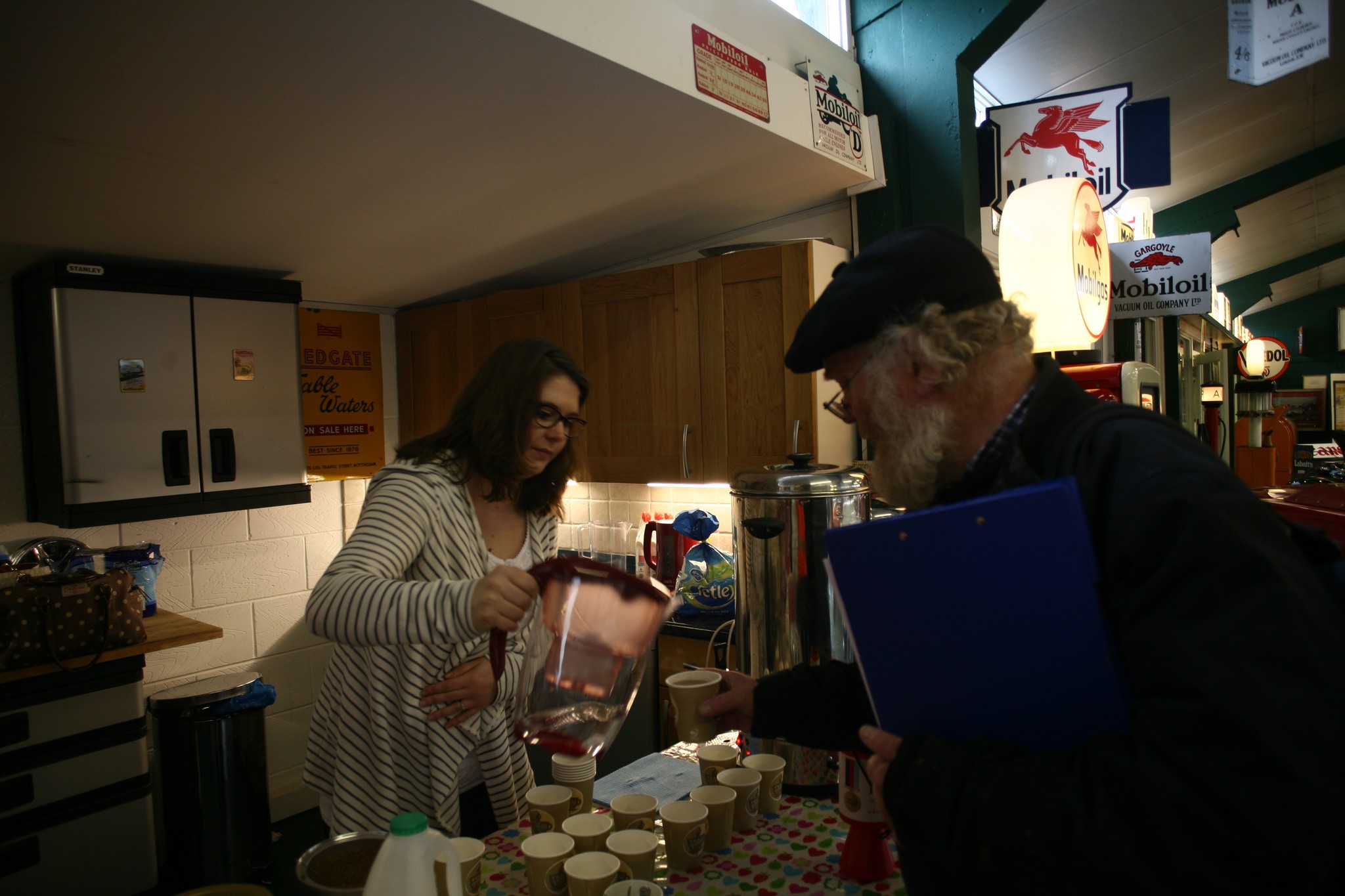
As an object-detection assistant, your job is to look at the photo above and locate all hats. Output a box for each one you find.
[785,234,1004,377]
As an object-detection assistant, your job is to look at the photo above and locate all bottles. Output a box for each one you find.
[358,813,461,896]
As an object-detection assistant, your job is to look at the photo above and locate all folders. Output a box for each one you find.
[819,478,1125,743]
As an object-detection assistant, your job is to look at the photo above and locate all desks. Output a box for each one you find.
[0,606,223,896]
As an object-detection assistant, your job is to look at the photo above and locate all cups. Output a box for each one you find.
[437,834,484,896]
[576,517,632,574]
[665,670,720,745]
[521,744,787,896]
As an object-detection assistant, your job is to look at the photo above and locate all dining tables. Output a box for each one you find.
[483,729,914,896]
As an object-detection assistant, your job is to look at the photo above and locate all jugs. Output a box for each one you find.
[486,559,673,760]
[641,518,700,590]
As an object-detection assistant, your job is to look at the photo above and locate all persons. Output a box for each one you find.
[664,227,1345,896]
[299,335,591,876]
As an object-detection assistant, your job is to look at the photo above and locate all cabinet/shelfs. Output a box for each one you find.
[398,241,861,483]
[0,669,163,896]
[10,257,312,529]
[658,612,735,752]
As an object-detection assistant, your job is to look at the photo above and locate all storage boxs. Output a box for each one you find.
[0,567,154,676]
[730,452,876,678]
[147,672,279,896]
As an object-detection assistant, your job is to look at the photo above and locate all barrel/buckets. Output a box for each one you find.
[731,452,880,802]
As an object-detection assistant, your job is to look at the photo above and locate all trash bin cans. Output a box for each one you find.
[145,671,279,896]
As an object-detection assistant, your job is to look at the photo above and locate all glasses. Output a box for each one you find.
[822,339,890,423]
[532,401,585,438]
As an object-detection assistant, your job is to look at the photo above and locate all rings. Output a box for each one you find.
[459,700,467,711]
